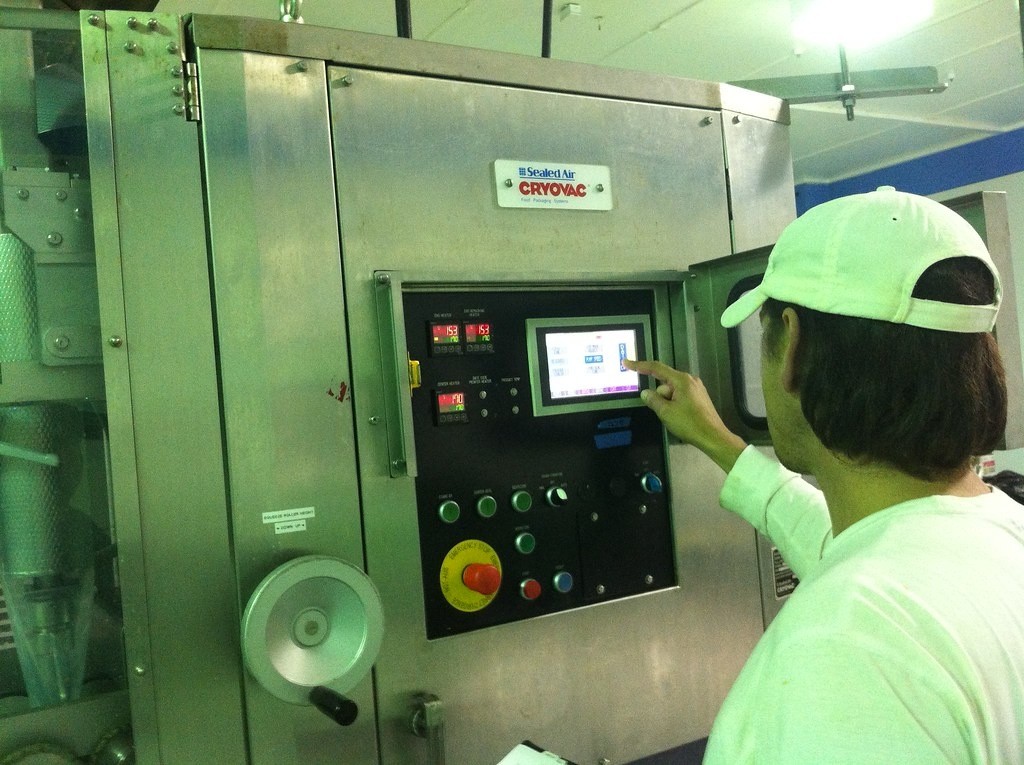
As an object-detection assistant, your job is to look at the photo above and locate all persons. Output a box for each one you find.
[620,186,1024,765]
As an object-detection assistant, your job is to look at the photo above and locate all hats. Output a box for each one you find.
[720,186,1003,332]
